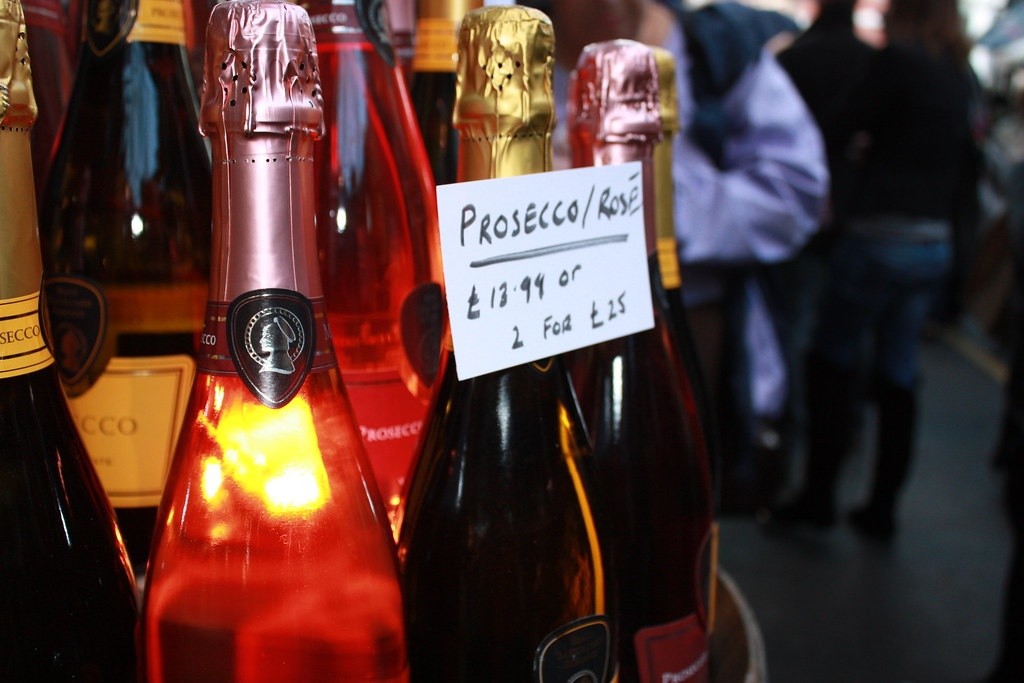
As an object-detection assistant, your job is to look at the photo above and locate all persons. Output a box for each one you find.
[551,1,1023,683]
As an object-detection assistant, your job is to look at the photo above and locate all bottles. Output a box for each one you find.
[0,0,713,683]
